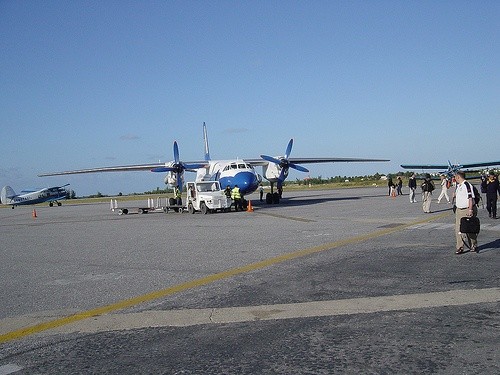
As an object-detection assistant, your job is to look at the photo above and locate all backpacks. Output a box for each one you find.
[446,180,451,189]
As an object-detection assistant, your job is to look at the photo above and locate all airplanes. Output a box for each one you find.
[37,122,391,205]
[0,184,77,209]
[400,160,500,189]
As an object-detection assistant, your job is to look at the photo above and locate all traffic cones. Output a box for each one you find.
[32,208,37,218]
[247,200,254,212]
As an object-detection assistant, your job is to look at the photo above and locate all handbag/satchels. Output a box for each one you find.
[459,217,481,234]
[481,182,486,193]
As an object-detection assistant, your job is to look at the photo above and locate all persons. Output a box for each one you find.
[422,174,434,213]
[437,174,456,203]
[452,172,477,254]
[224,185,243,212]
[259,184,264,201]
[188,186,193,198]
[175,185,182,205]
[481,172,500,219]
[388,177,403,197]
[408,173,417,203]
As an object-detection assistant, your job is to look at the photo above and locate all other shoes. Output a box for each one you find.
[489,213,491,217]
[493,216,496,219]
[455,249,463,254]
[470,246,476,252]
[410,201,418,203]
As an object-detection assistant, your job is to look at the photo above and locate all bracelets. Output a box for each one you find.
[469,210,473,211]
